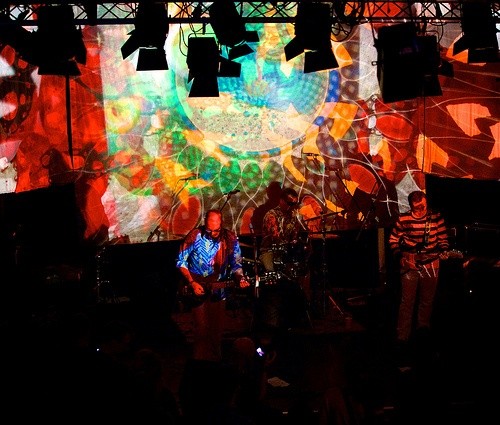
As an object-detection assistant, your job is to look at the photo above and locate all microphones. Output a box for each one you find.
[325,166,342,171]
[224,190,240,195]
[181,177,200,181]
[303,152,319,156]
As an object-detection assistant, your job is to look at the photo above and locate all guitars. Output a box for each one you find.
[411,250,464,265]
[203,268,304,295]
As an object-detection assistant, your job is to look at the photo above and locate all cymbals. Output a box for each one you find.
[237,232,278,247]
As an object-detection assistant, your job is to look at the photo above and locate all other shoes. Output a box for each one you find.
[396,335,407,345]
[419,320,430,330]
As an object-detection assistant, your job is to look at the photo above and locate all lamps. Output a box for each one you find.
[0,0,500,104]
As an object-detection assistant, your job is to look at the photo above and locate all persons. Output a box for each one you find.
[387,190,450,375]
[174,209,251,363]
[261,187,311,329]
[0,312,500,425]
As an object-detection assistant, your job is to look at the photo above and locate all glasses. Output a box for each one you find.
[206,224,221,233]
[286,200,294,206]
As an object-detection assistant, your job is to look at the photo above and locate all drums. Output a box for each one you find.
[271,243,310,280]
